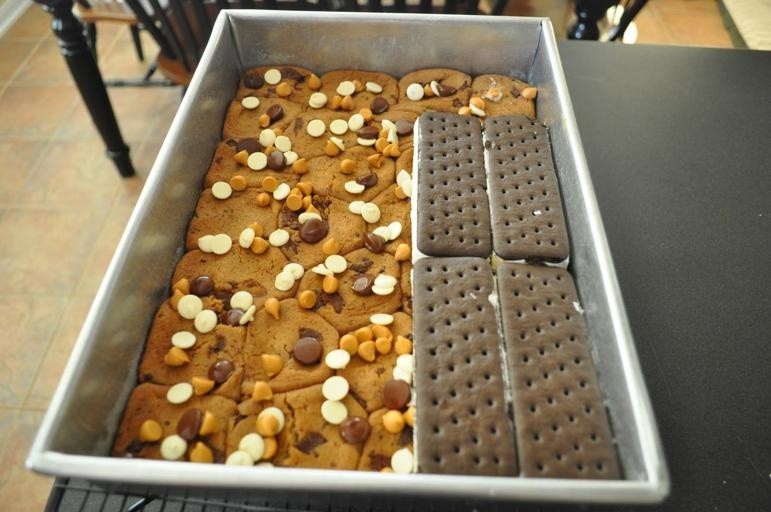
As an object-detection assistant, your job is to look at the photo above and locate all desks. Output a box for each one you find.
[32,0,173,176]
[39,37,770,510]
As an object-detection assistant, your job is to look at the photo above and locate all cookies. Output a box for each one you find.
[107,66,624,478]
